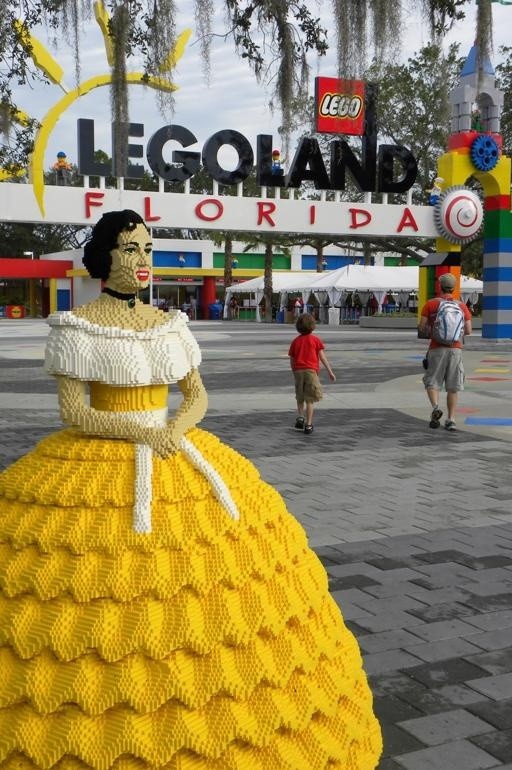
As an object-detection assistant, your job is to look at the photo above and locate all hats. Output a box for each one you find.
[439,273,455,290]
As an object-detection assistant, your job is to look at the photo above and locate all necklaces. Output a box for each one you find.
[102,288,136,308]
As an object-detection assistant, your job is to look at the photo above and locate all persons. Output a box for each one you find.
[0,210,384,769]
[230,298,237,319]
[418,273,472,430]
[288,313,336,434]
[353,294,361,311]
[294,298,302,322]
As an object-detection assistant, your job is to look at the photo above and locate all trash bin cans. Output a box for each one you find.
[209,303,220,319]
[276,311,284,323]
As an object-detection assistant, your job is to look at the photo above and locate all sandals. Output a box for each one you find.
[295,416,314,433]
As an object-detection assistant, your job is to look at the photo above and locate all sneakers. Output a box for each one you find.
[446,419,456,432]
[429,410,442,429]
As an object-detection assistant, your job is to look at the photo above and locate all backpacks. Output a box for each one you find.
[431,297,466,345]
